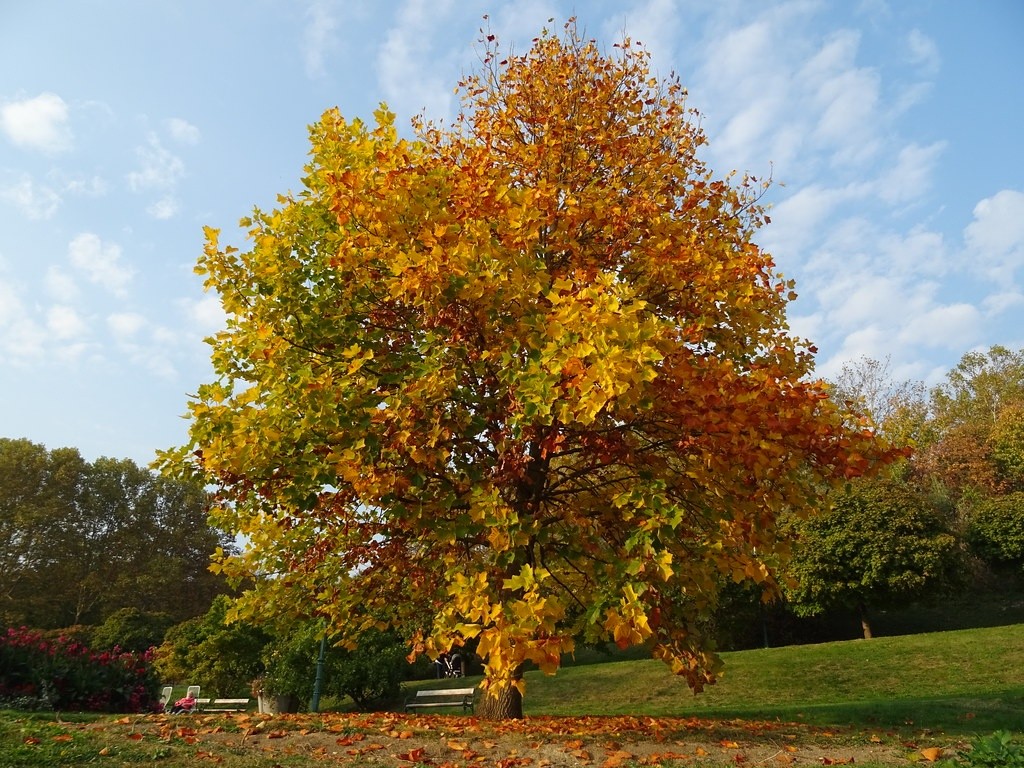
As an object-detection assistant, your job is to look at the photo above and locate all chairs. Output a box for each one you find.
[176,686,201,715]
[148,687,173,714]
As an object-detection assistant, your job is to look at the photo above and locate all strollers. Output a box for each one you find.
[435,653,466,680]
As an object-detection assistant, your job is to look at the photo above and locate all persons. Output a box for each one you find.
[434,653,445,678]
[165,690,195,715]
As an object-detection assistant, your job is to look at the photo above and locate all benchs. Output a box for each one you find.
[406,688,478,714]
[190,698,251,714]
